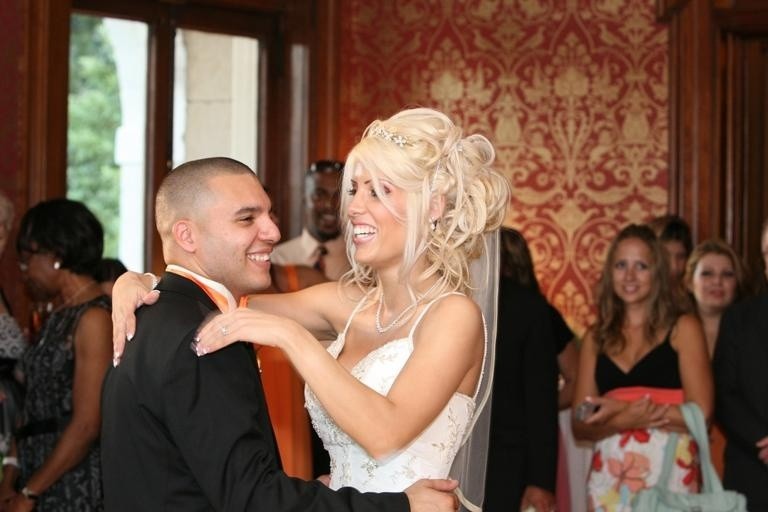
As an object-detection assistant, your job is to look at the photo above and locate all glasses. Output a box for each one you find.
[309,160,346,174]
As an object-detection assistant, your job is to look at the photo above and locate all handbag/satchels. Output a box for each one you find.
[631,401,750,512]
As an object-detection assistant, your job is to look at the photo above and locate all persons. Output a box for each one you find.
[485,215,767,512]
[1,197,127,511]
[109,102,512,511]
[96,157,460,510]
[268,160,351,281]
[255,185,327,481]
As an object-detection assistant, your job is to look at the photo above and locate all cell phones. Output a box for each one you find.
[577,402,599,421]
